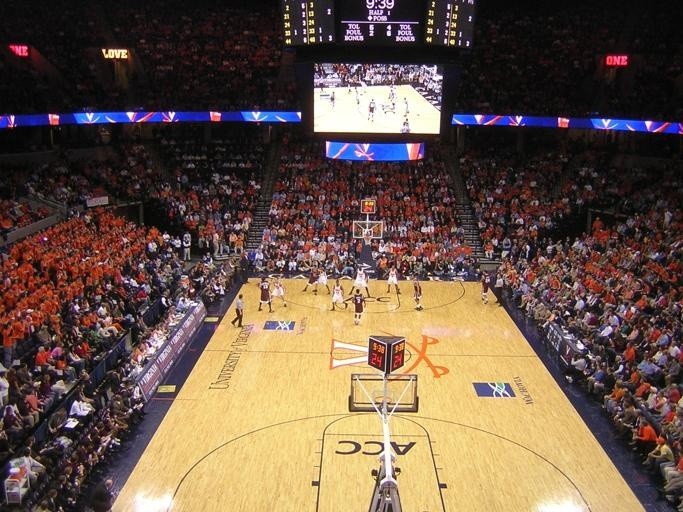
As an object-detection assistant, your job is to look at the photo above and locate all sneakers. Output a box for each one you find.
[495,300,503,307]
[397,291,401,294]
[481,296,488,304]
[616,434,657,476]
[345,303,348,308]
[331,308,335,311]
[231,322,243,327]
[658,482,683,512]
[518,304,532,318]
[416,305,422,311]
[386,290,390,293]
[354,319,360,325]
[258,303,287,312]
[566,375,574,383]
[303,288,330,295]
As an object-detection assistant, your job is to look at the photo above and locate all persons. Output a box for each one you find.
[1,0,683,512]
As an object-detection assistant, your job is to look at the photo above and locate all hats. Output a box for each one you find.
[656,436,664,443]
